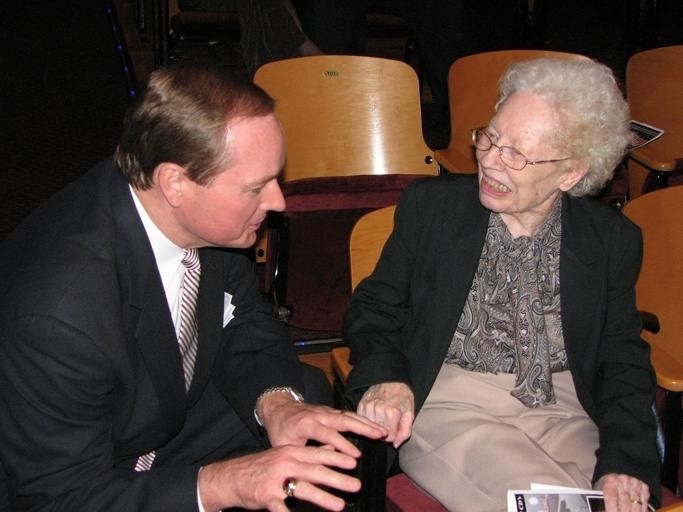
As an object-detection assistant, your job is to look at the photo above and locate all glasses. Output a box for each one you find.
[470,123,575,169]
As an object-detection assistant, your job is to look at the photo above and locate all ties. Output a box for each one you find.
[179,248,202,389]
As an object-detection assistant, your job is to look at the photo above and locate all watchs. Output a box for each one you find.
[253,383,305,428]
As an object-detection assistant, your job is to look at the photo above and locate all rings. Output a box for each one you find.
[628,496,641,505]
[281,475,299,497]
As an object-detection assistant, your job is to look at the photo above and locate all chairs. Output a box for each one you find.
[622,46,682,199]
[621,184,682,398]
[252,52,443,326]
[433,48,596,176]
[326,203,401,512]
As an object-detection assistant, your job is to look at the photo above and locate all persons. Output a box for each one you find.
[0,52,387,512]
[327,53,669,512]
[233,1,326,82]
